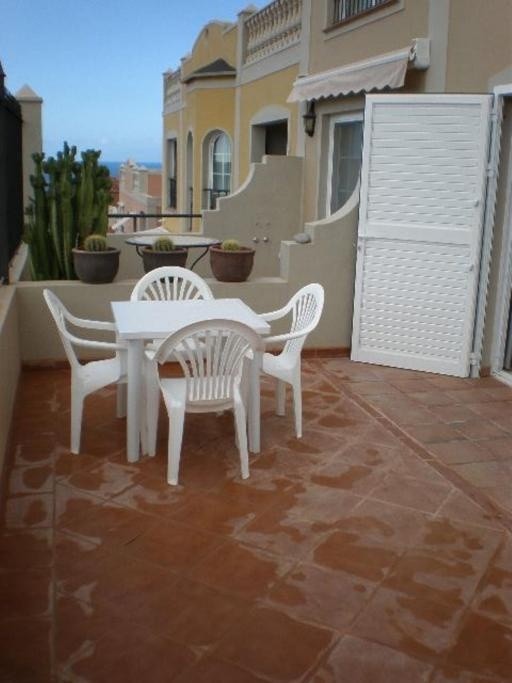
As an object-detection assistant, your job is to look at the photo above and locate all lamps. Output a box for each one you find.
[303,102,316,137]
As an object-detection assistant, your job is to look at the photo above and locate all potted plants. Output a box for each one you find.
[72,236,121,283]
[210,240,256,282]
[141,237,187,282]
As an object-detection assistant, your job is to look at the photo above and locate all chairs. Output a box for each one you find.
[220,281,325,453]
[142,317,263,486]
[128,267,225,418]
[43,288,161,456]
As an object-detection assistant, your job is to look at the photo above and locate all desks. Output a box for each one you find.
[109,298,271,464]
[126,235,221,271]
